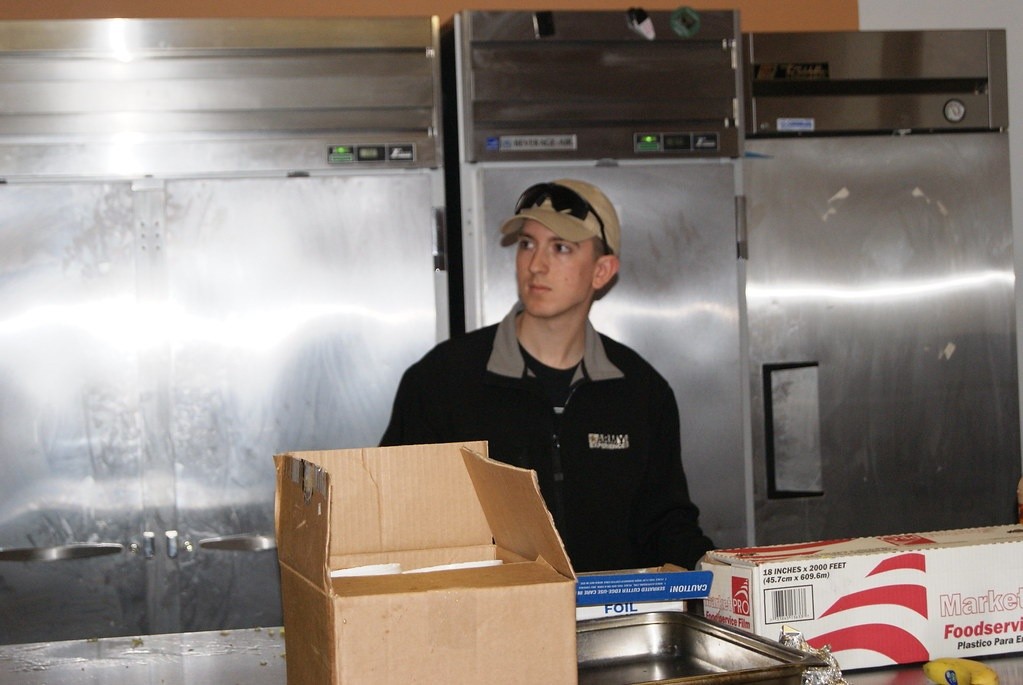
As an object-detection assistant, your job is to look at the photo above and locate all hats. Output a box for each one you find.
[501,179,621,254]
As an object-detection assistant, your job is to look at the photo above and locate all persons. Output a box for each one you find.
[378,178,718,570]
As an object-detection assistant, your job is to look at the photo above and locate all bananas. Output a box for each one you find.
[923,657,999,685]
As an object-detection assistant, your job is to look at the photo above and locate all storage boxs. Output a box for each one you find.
[703,525,1023,672]
[575,563,714,620]
[273,440,578,684]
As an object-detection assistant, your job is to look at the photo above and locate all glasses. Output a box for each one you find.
[514,182,609,254]
